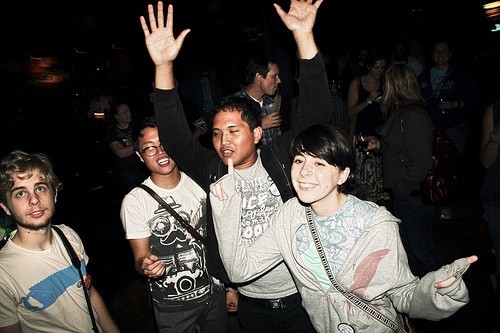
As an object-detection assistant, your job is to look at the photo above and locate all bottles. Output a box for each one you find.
[438,89,448,120]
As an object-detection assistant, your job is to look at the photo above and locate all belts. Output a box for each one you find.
[151,286,220,312]
[237,292,302,310]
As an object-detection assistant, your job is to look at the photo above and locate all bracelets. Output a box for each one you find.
[367,98,372,104]
[226,288,238,293]
[456,100,461,108]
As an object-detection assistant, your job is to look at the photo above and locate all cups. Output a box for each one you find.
[265,103,278,115]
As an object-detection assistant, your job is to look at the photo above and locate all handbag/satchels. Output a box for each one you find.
[420,170,457,205]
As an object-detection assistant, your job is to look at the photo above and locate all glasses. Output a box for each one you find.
[138,144,165,157]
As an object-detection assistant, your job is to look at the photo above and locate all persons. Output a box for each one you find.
[233,57,299,146]
[209,124,478,333]
[290,42,425,133]
[0,150,120,333]
[347,56,386,139]
[107,103,150,176]
[140,0,331,333]
[416,43,478,218]
[120,115,238,333]
[481,101,500,295]
[364,63,442,272]
